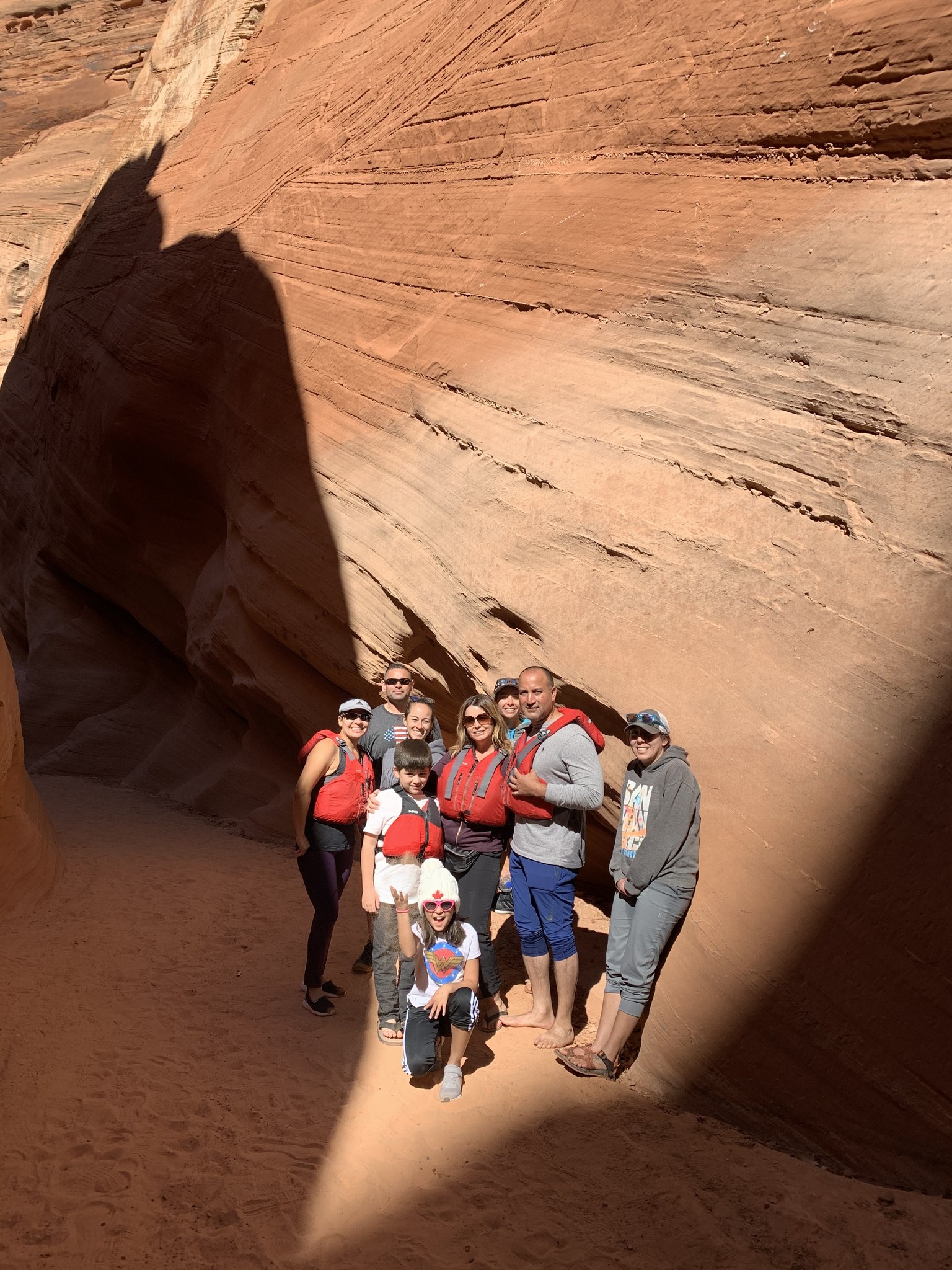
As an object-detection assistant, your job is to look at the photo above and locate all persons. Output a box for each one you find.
[294,663,604,1050]
[390,858,480,1102]
[555,710,700,1082]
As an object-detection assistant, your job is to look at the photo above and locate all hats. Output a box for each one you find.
[339,699,373,716]
[417,857,460,915]
[624,709,670,735]
[494,678,519,695]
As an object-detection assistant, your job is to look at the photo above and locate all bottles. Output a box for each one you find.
[498,877,512,893]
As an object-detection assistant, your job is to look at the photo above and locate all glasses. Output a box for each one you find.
[406,695,435,710]
[384,678,411,685]
[626,713,667,732]
[340,712,372,722]
[463,712,492,727]
[421,900,454,912]
[493,678,519,698]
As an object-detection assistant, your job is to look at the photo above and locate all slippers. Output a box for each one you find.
[525,973,533,994]
[475,985,484,1000]
[480,1010,507,1033]
[376,1019,406,1046]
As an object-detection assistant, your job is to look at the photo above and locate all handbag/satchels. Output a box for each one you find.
[443,842,481,872]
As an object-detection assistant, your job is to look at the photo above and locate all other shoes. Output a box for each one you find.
[352,942,375,973]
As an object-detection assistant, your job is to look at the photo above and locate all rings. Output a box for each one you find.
[513,785,516,790]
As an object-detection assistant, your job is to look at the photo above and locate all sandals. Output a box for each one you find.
[563,1050,619,1082]
[553,1043,595,1060]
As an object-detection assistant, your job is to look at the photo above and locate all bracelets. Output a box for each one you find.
[396,908,410,912]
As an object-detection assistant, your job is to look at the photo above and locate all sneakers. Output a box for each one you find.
[301,979,348,998]
[490,890,515,914]
[438,1064,462,1102]
[303,992,336,1016]
[430,1035,445,1072]
